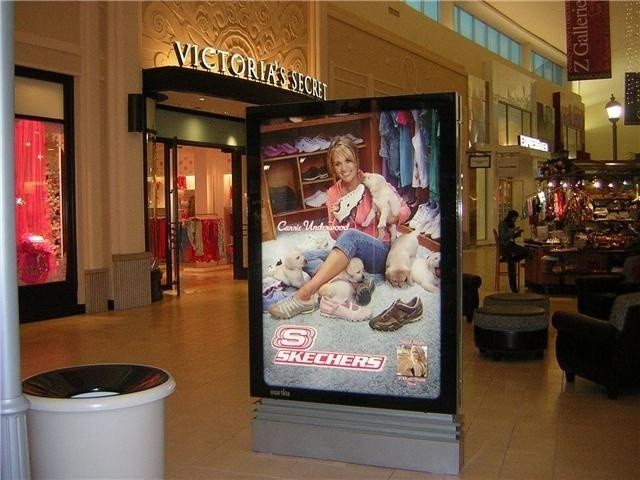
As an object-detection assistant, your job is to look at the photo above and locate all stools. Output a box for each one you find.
[462,273,482,323]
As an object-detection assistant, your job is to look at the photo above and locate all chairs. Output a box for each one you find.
[551,257,640,401]
[492,228,520,292]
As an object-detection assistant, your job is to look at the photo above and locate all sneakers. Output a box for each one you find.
[295,136,331,153]
[262,280,315,320]
[319,297,372,322]
[261,143,299,157]
[499,257,507,262]
[304,187,331,208]
[511,287,516,292]
[403,196,441,240]
[357,277,375,306]
[369,296,424,332]
[346,133,364,145]
[301,167,329,180]
[332,184,365,225]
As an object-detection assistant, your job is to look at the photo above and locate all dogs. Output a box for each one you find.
[318,280,357,302]
[411,251,441,294]
[361,173,401,229]
[262,249,307,289]
[294,234,329,252]
[384,219,430,289]
[311,257,365,304]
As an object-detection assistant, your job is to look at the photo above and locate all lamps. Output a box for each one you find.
[604,94,622,159]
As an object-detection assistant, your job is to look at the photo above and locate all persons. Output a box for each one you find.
[624,185,640,216]
[267,135,411,321]
[405,347,428,379]
[498,210,529,293]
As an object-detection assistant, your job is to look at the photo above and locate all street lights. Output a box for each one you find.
[605,92,625,159]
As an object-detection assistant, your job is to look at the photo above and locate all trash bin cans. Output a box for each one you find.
[151,270,163,302]
[22,363,176,480]
